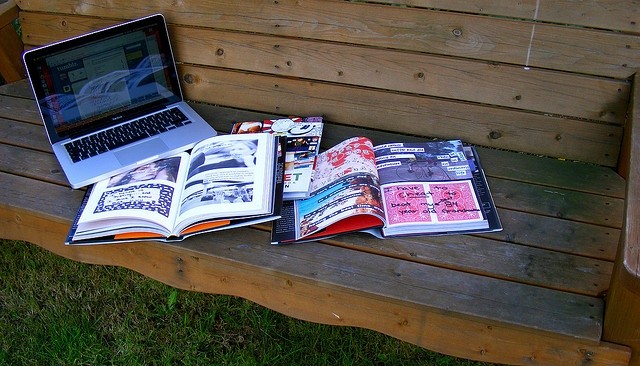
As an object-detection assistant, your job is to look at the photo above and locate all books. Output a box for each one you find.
[41,64,167,123]
[229,116,325,201]
[271,139,504,247]
[63,132,280,247]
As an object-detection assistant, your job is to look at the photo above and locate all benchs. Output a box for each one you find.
[1,1,640,365]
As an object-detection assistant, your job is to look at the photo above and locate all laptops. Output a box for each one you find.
[22,13,218,191]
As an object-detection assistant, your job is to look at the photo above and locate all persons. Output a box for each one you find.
[108,161,175,186]
[222,141,256,167]
